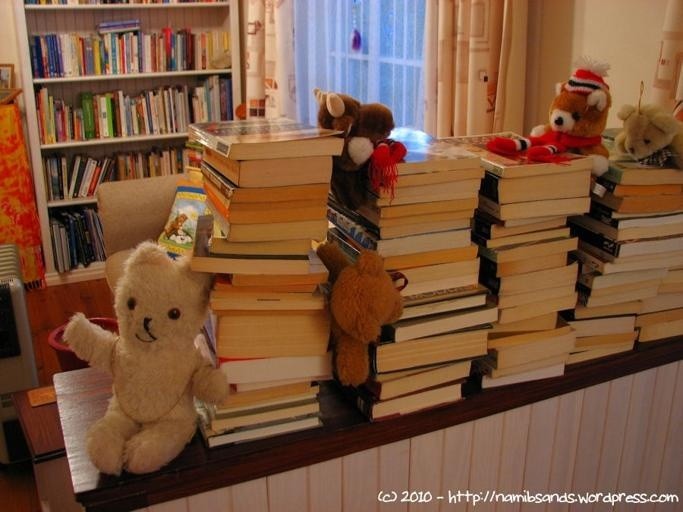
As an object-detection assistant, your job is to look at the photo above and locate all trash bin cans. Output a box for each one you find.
[47,317,119,372]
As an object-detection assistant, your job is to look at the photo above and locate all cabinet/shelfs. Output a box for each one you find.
[12,1,245,279]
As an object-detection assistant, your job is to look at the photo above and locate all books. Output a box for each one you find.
[23,19,239,279]
[155,114,683,458]
[25,0,237,6]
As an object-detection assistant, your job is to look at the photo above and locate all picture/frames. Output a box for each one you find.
[0,64,23,105]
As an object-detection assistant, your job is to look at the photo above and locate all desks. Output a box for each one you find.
[49,334,683,512]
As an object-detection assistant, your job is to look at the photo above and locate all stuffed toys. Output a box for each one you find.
[609,104,683,171]
[482,54,614,191]
[311,86,407,210]
[60,238,234,474]
